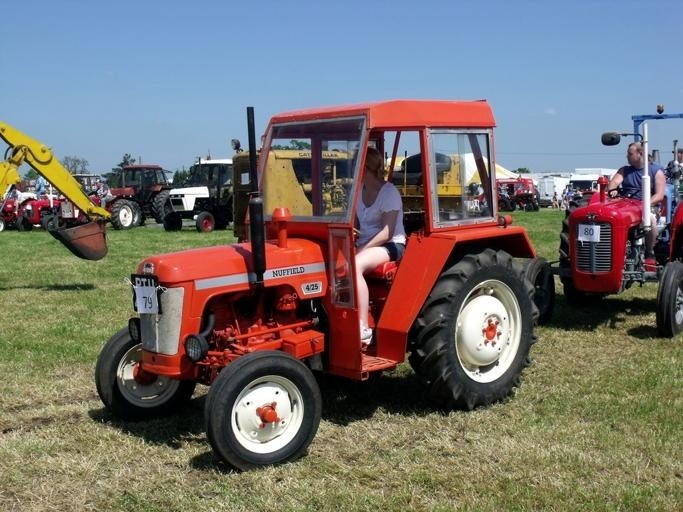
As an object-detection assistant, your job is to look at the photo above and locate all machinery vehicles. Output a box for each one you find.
[519,104,683,339]
[94,97,541,473]
[0,121,112,261]
[0,138,618,233]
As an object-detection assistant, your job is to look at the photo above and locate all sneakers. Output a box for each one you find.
[360,321,373,344]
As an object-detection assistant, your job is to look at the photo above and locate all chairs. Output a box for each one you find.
[367,214,428,278]
[655,188,669,241]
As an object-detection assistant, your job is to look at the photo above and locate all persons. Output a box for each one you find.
[608,142,683,259]
[95,180,112,209]
[10,186,25,216]
[344,146,407,349]
[33,173,45,201]
[552,189,582,209]
[496,182,511,200]
[470,183,489,208]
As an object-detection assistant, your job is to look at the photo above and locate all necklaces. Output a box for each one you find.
[359,180,386,207]
